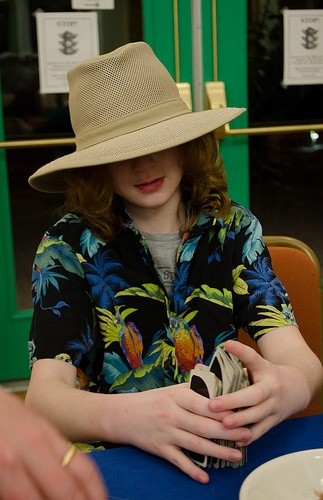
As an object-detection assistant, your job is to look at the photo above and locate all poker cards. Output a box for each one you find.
[181,346,251,468]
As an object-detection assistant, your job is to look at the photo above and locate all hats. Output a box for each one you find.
[28,42,246,192]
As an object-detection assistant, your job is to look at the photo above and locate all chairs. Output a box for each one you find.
[236,237,323,419]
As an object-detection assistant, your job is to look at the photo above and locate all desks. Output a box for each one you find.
[82,415,323,500]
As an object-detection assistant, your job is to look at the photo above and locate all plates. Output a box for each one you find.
[239,448,323,500]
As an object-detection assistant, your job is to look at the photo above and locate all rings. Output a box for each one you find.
[62,443,78,467]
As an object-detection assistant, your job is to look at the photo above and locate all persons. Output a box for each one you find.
[21,40,323,485]
[1,385,107,500]
